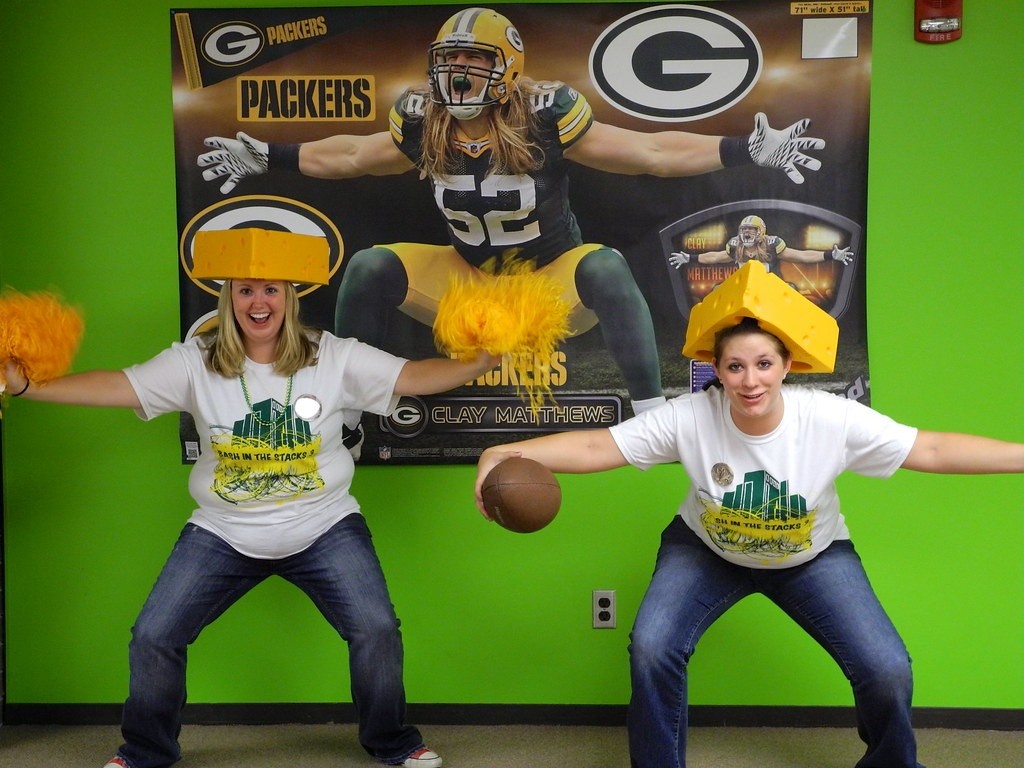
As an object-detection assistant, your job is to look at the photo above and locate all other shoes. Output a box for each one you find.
[341,423,364,462]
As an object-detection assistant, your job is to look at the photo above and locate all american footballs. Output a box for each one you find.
[481,457,561,534]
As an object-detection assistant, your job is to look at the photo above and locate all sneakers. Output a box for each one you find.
[102,753,132,768]
[400,748,442,767]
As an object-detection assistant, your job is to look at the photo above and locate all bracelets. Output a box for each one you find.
[12,379,29,397]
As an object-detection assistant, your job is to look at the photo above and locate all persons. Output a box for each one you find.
[669,215,854,293]
[475,317,1024,768]
[197,9,824,462]
[0,278,501,767]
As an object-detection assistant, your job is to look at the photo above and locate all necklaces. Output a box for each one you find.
[239,374,293,425]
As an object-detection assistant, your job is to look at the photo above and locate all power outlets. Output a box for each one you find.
[593,590,616,629]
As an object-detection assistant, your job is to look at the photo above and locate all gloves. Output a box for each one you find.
[719,110,826,186]
[828,245,854,266]
[669,251,698,270]
[195,131,301,194]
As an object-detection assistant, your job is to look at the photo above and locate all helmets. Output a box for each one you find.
[426,8,525,105]
[740,214,766,243]
